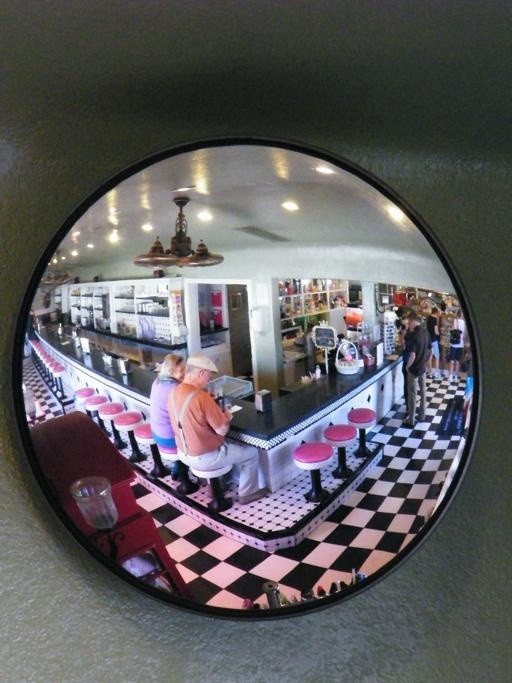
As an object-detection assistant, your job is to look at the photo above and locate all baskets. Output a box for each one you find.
[335,341,360,374]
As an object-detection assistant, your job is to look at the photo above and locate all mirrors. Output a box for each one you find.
[14,136,482,623]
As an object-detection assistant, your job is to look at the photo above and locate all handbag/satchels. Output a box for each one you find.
[448,328,462,344]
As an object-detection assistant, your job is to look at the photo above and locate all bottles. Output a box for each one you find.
[279,277,305,296]
[240,569,366,608]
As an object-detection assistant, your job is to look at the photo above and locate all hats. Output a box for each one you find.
[186,354,219,373]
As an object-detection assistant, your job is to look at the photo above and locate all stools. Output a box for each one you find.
[323,424,357,478]
[29,340,232,514]
[294,442,334,502]
[348,408,377,458]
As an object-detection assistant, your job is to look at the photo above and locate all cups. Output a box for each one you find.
[117,356,133,375]
[209,318,215,328]
[67,475,119,529]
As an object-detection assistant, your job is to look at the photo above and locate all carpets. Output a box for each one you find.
[435,398,466,436]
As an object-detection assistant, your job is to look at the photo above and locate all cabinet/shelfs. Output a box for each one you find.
[67,277,187,345]
[29,412,193,600]
[53,284,67,314]
[279,278,350,342]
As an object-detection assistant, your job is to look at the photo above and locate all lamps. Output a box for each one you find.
[71,475,125,561]
[42,251,71,285]
[132,197,223,267]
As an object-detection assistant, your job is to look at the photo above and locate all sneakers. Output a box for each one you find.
[206,478,226,498]
[415,413,426,421]
[403,418,414,426]
[238,486,268,504]
[170,459,200,484]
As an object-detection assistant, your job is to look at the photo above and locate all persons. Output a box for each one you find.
[395,303,466,428]
[149,355,187,482]
[166,354,269,504]
